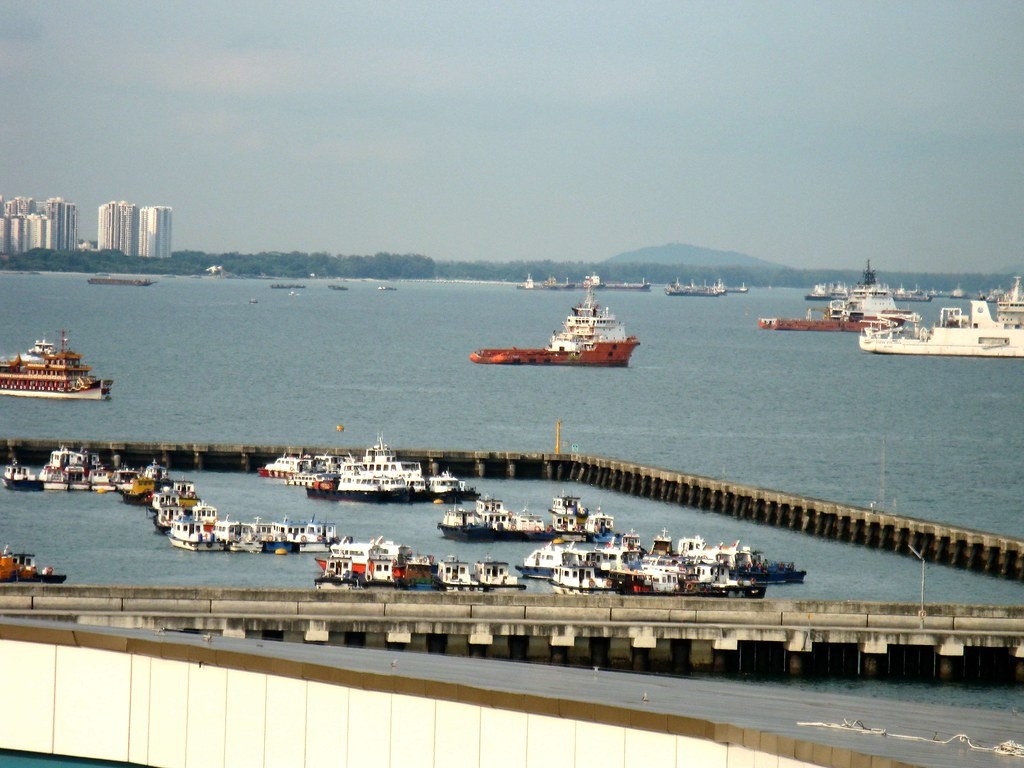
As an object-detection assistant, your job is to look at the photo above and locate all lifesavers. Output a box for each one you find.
[589,581,595,587]
[290,466,294,471]
[317,535,323,541]
[300,535,306,542]
[750,579,756,585]
[267,535,273,541]
[535,525,541,532]
[281,536,287,541]
[562,524,566,530]
[737,578,743,585]
[606,580,612,586]
[511,524,516,530]
[686,583,692,590]
[576,524,581,530]
[508,510,513,516]
[546,527,551,532]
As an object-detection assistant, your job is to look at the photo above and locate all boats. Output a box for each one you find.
[804,282,1005,303]
[517,272,750,298]
[857,274,1024,358]
[469,276,641,369]
[756,258,912,332]
[0,329,113,401]
[87,272,159,286]
[0,429,807,599]
